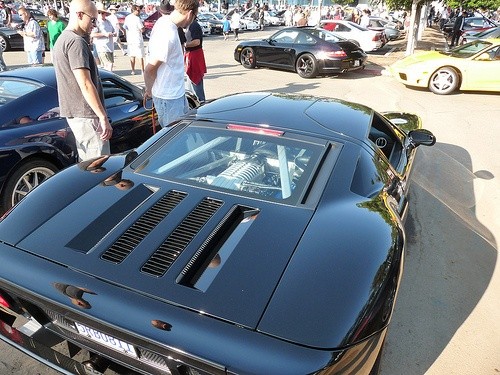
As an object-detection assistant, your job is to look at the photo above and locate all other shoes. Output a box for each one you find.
[224,36,227,40]
[235,37,239,41]
[130,71,135,75]
[123,51,127,56]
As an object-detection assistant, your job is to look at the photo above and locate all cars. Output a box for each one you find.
[346,5,403,41]
[440,15,500,52]
[283,19,388,54]
[182,5,287,37]
[390,38,500,95]
[112,10,161,40]
[0,8,50,52]
[0,64,201,218]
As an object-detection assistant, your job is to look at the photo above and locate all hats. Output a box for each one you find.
[97,4,111,16]
[156,0,174,14]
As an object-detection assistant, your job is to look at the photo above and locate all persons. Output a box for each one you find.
[52,0,113,162]
[16,6,46,64]
[47,9,65,63]
[184,20,207,102]
[231,9,240,41]
[451,12,468,48]
[0,45,6,67]
[123,5,145,75]
[0,1,12,24]
[90,3,127,72]
[321,6,407,29]
[144,0,199,129]
[156,0,187,56]
[427,4,500,28]
[200,0,312,31]
[222,16,230,40]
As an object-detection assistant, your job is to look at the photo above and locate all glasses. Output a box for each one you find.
[75,11,97,23]
[190,9,197,20]
[20,12,25,16]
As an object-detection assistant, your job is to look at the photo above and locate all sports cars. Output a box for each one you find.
[0,90,436,375]
[234,25,368,80]
